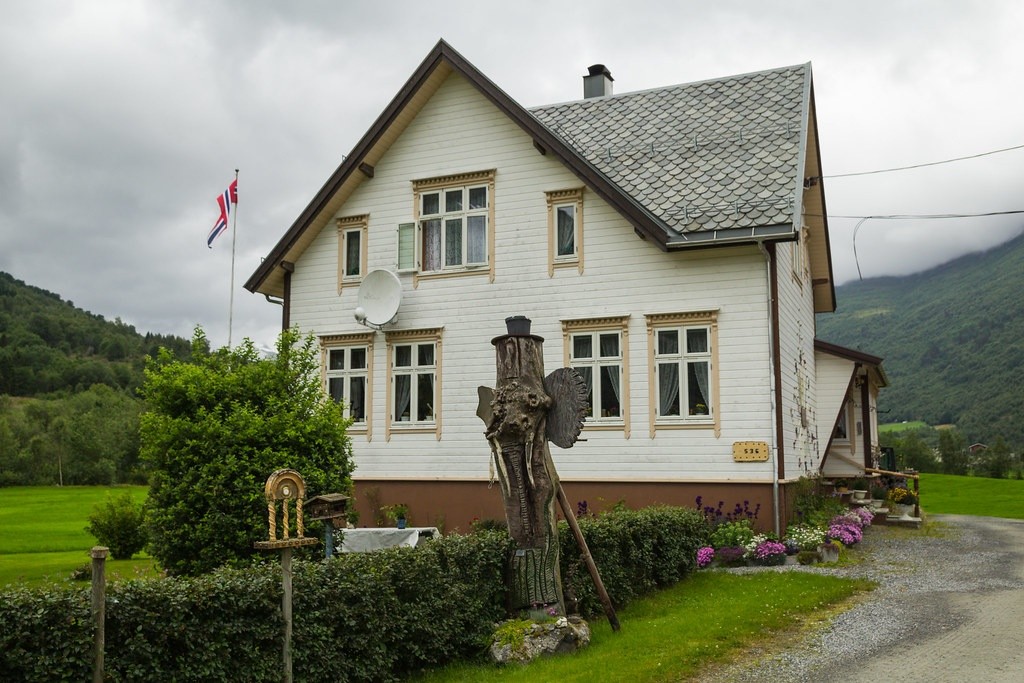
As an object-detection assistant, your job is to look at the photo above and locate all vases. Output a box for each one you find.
[895,504,916,516]
[696,412,705,415]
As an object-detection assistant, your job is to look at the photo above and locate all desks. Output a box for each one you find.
[317,526,440,552]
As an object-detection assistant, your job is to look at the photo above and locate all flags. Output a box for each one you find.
[206,179,238,249]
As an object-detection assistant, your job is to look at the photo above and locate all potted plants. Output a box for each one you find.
[871,486,886,508]
[401,412,410,422]
[382,504,409,529]
[835,477,849,492]
[425,410,433,419]
[852,476,869,500]
[817,538,843,563]
[345,508,359,529]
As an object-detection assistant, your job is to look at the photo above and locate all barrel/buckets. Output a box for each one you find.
[505,316,531,335]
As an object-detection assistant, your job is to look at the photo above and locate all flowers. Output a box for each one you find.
[889,488,920,505]
[609,407,618,414]
[694,403,707,412]
